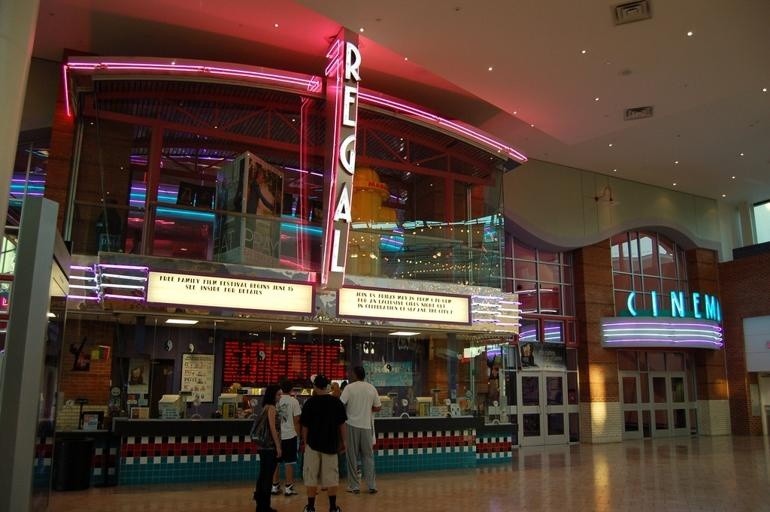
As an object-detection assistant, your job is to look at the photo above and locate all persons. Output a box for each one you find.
[338,360,382,494]
[254,382,283,512]
[340,380,347,396]
[328,382,342,399]
[271,378,302,496]
[99,199,122,231]
[230,382,253,418]
[181,186,192,204]
[298,374,348,512]
[245,167,274,249]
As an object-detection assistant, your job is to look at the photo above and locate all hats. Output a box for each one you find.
[310,374,327,387]
[281,379,298,392]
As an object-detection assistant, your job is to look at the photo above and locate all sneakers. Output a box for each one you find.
[329,506,341,512]
[271,481,283,496]
[343,489,360,496]
[369,489,378,494]
[303,504,315,512]
[284,482,299,496]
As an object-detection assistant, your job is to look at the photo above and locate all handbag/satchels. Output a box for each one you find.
[250,404,277,448]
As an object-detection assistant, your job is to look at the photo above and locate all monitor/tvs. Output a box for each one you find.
[416,397,434,412]
[158,394,183,414]
[378,395,394,408]
[218,393,239,407]
